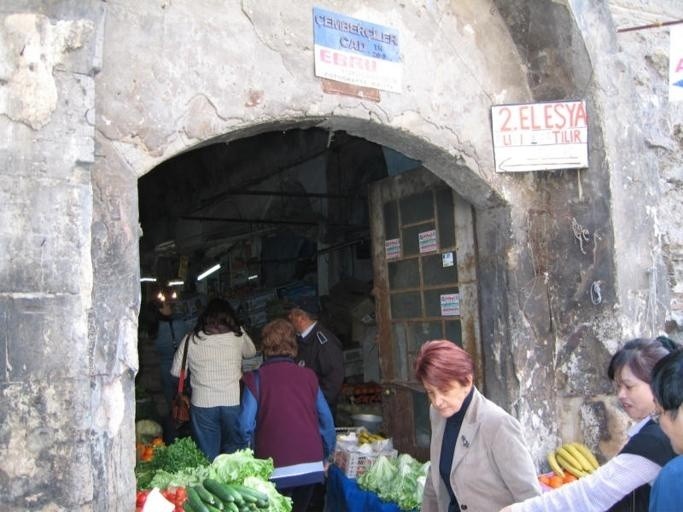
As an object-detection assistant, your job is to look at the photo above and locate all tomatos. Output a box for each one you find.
[136,487,186,512]
[337,384,382,405]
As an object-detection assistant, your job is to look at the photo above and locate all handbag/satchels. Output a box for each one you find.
[170,393,190,423]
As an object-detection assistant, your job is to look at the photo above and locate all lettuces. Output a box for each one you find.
[359,454,433,511]
[146,448,292,512]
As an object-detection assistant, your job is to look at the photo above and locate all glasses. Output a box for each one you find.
[649,408,670,424]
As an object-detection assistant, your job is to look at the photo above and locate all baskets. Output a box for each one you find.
[333,450,398,479]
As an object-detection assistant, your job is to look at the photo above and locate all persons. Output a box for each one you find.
[416,339,542,512]
[169,299,257,464]
[499,336,683,512]
[240,296,344,512]
[155,297,194,444]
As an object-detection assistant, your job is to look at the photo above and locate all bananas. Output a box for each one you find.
[359,429,383,445]
[547,441,597,478]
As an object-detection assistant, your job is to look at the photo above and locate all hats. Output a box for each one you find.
[282,296,320,313]
